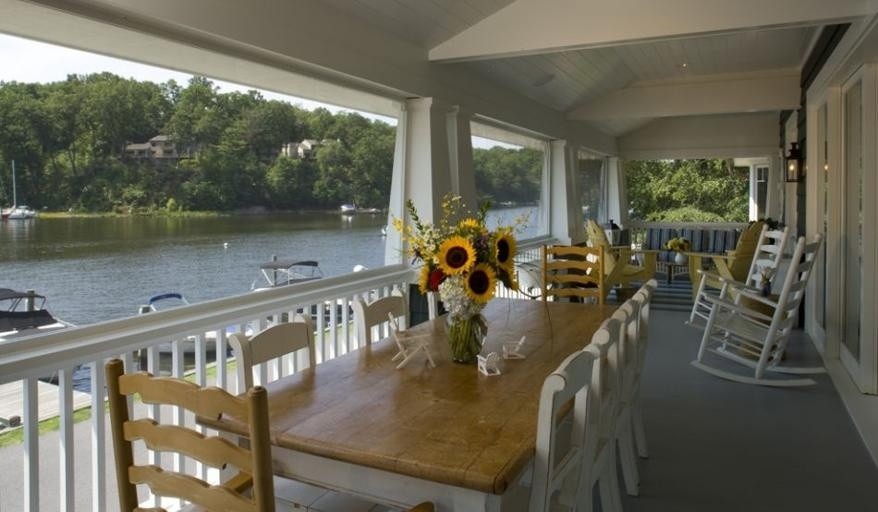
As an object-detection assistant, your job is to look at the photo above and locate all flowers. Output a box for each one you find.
[390,191,564,356]
[664,237,693,253]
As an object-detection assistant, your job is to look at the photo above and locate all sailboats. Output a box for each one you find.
[2,159,36,219]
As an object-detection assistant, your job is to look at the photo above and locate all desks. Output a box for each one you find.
[195,297,621,512]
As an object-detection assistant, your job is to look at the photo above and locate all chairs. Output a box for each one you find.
[681,221,829,388]
[351,289,410,348]
[582,218,660,304]
[105,358,435,512]
[540,244,605,304]
[503,278,659,512]
[476,352,501,376]
[228,313,316,395]
[502,334,529,361]
[388,312,437,369]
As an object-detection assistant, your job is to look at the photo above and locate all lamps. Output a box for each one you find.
[782,142,806,182]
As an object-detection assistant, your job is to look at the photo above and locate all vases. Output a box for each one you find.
[443,314,489,365]
[675,251,688,265]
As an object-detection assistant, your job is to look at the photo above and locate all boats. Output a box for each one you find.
[0,207,15,221]
[339,204,355,215]
[249,257,354,318]
[0,287,83,386]
[133,292,254,353]
[381,224,388,236]
